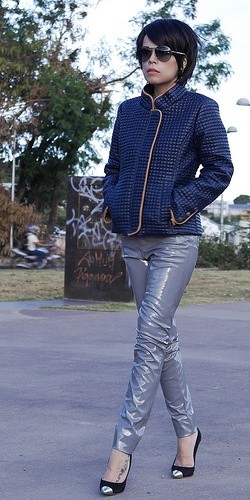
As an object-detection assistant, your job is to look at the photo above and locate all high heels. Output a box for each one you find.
[101,445,131,495]
[171,428,203,479]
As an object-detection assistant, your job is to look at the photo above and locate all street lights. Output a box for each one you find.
[220,126,238,243]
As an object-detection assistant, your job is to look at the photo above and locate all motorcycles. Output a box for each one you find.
[10,241,52,270]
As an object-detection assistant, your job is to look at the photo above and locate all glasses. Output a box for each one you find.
[136,45,186,63]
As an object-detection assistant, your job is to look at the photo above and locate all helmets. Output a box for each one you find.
[27,225,40,235]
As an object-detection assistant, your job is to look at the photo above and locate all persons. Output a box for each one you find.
[26,224,49,268]
[100,19,234,495]
